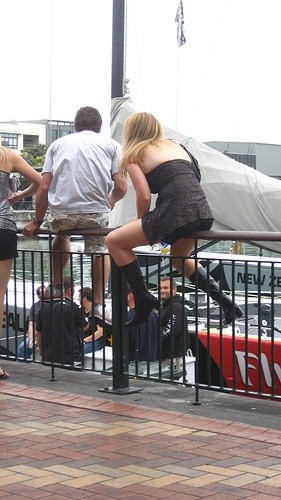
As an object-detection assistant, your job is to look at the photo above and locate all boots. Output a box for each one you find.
[116,258,161,328]
[189,265,244,326]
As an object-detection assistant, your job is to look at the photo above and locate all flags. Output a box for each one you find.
[175,0,186,48]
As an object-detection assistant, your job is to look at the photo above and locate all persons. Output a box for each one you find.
[123,283,156,362]
[79,285,112,344]
[24,106,128,330]
[38,278,86,363]
[105,113,245,328]
[17,287,42,359]
[0,136,42,379]
[156,274,186,364]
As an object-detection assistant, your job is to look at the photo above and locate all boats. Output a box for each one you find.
[5,278,281,399]
[10,328,197,385]
[128,244,281,295]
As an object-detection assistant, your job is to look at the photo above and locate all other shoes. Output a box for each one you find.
[0,369,9,379]
